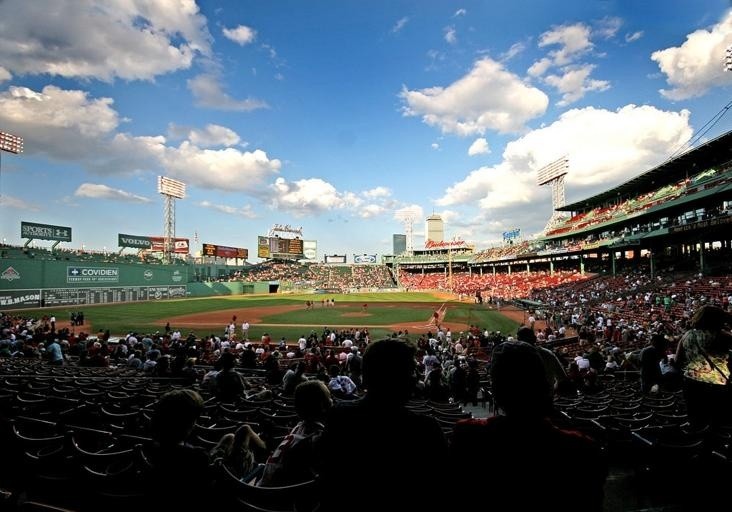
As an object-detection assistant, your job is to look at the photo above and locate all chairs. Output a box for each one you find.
[0,266,732,512]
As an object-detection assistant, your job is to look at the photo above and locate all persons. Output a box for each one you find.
[302,297,336,312]
[475,293,539,310]
[205,236,539,293]
[539,164,731,309]
[1,310,732,511]
